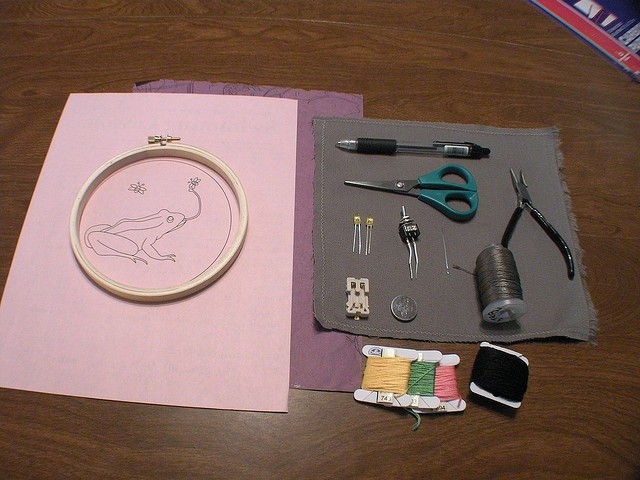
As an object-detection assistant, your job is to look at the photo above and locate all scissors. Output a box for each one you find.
[344,164,479,224]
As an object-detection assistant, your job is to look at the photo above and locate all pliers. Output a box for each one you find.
[502,169,575,281]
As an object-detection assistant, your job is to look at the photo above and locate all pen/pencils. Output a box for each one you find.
[334,138,490,159]
